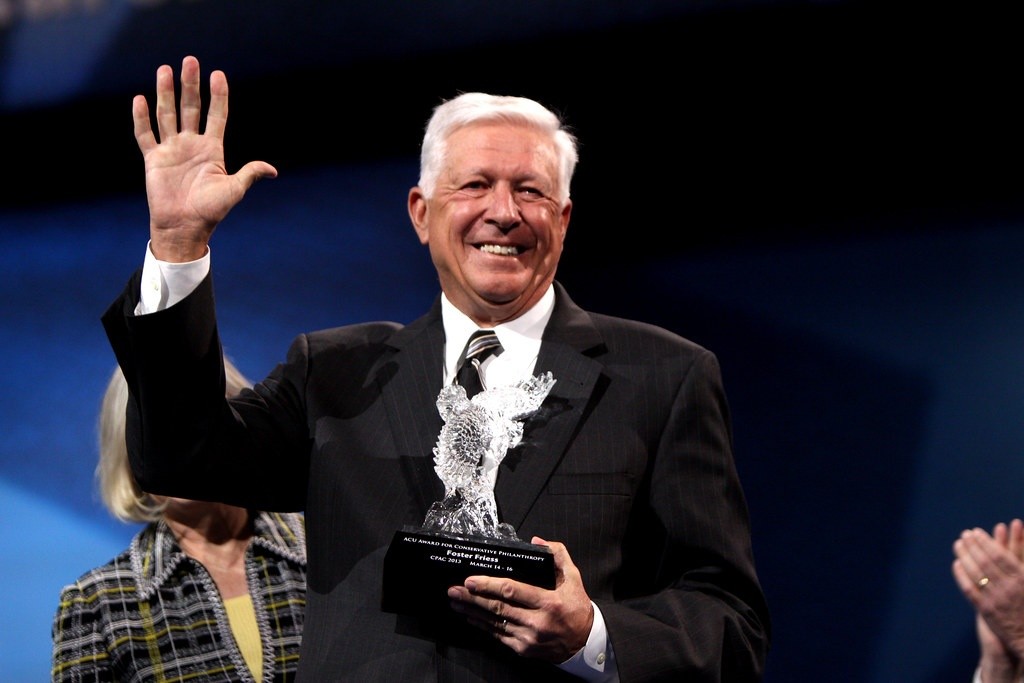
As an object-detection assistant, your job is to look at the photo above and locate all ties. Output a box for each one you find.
[449,330,500,479]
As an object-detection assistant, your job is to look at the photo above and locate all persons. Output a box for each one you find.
[952,519,1024,683]
[101,55,770,683]
[48,337,314,683]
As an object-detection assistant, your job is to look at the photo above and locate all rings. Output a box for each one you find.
[501,619,507,630]
[977,577,989,589]
